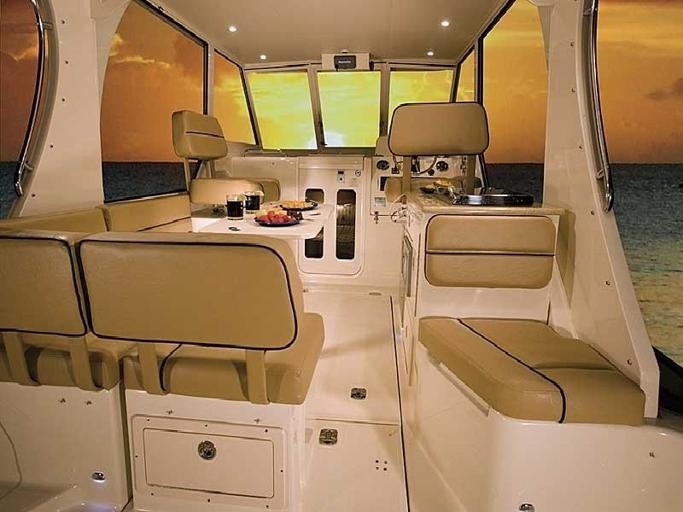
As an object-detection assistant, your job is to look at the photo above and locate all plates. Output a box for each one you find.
[279,200,318,211]
[253,216,299,227]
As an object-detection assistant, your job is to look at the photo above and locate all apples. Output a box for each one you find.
[260,211,291,224]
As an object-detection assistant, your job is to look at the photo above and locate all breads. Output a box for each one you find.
[282,200,308,208]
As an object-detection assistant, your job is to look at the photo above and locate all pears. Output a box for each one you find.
[256,208,266,219]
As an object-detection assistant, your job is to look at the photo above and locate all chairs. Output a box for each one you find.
[169,107,280,206]
[415,213,645,428]
[384,101,488,202]
[1,194,326,402]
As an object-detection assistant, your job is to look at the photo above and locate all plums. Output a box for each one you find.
[287,210,302,220]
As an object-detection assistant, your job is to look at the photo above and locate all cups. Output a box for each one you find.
[243,190,262,215]
[225,192,244,219]
